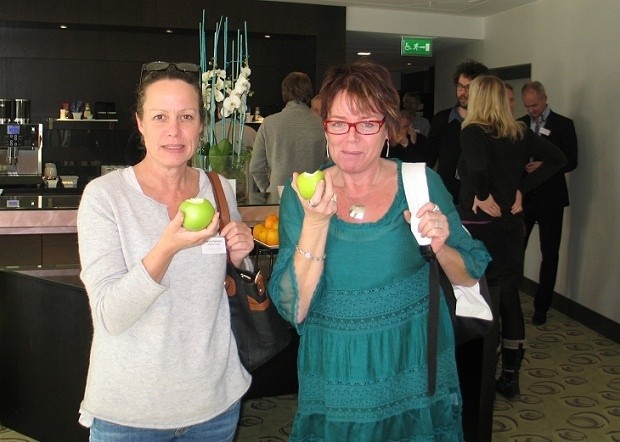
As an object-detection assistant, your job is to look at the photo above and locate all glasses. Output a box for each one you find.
[138,61,202,90]
[457,82,470,92]
[323,117,386,135]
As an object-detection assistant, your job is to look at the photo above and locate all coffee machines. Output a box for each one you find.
[0,123,43,187]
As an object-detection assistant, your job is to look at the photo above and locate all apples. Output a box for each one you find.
[297,169,325,201]
[178,197,215,232]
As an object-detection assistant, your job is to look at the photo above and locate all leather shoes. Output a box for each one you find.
[532,307,547,326]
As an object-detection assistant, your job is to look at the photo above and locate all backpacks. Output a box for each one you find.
[401,161,500,396]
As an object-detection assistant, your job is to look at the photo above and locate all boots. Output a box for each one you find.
[494,343,525,400]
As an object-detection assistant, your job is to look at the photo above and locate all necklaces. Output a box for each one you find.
[337,166,381,222]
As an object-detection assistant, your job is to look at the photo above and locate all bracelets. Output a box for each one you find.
[294,244,326,263]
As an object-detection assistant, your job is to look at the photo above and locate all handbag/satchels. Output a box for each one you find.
[209,262,294,373]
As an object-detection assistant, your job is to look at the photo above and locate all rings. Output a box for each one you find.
[330,197,337,204]
[435,220,443,229]
[308,200,315,208]
[433,203,439,214]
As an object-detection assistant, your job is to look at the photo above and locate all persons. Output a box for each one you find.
[266,58,491,440]
[450,74,527,403]
[200,100,258,157]
[76,60,257,441]
[428,61,490,172]
[514,81,580,323]
[248,70,329,207]
[503,83,516,118]
[307,94,325,118]
[399,93,434,136]
[380,109,432,164]
